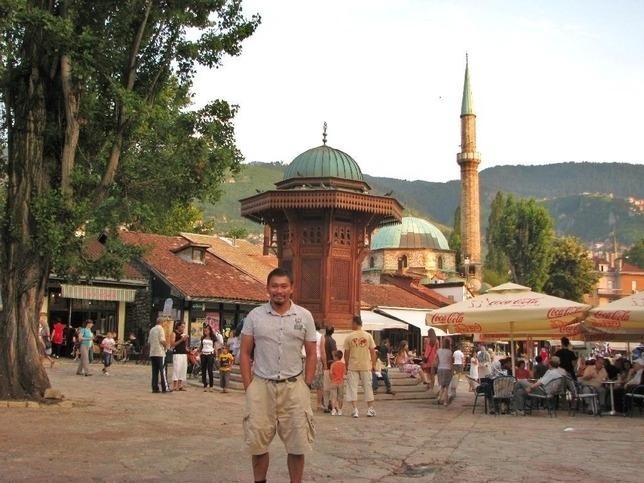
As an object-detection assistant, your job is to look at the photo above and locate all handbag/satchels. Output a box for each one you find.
[421,355,427,362]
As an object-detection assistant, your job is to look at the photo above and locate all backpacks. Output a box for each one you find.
[73,327,85,348]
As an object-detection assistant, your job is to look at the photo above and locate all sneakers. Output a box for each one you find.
[367,408,377,417]
[332,409,337,416]
[103,371,110,377]
[351,408,359,418]
[386,391,395,394]
[338,409,342,415]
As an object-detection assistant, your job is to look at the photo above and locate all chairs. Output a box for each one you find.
[465,374,644,418]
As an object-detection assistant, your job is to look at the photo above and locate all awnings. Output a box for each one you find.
[380,309,461,336]
[360,310,408,331]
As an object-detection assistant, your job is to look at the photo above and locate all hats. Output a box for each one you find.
[632,359,644,366]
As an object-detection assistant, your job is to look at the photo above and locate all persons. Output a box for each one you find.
[147,317,173,393]
[197,324,217,392]
[215,329,233,393]
[373,339,421,394]
[38,315,78,367]
[469,337,644,415]
[100,332,116,376]
[172,320,189,392]
[232,265,319,483]
[76,319,97,376]
[343,317,376,418]
[424,329,464,407]
[301,323,346,415]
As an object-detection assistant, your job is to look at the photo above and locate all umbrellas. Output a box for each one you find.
[423,280,591,382]
[587,291,644,344]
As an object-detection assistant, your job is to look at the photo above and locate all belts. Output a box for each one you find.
[252,370,304,384]
